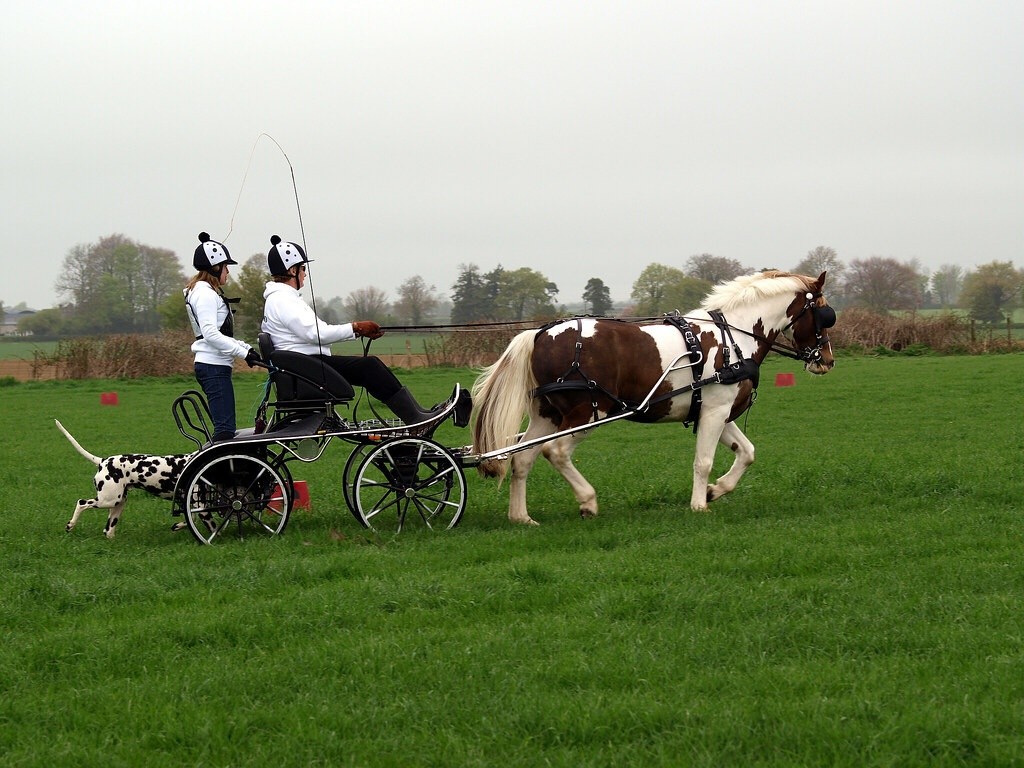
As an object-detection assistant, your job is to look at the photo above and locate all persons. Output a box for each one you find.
[261,235,447,435]
[182,232,262,441]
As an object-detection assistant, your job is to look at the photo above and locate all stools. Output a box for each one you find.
[266,478,309,514]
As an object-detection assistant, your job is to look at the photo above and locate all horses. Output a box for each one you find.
[466,267,836,530]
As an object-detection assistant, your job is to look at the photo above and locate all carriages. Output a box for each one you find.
[170,267,838,544]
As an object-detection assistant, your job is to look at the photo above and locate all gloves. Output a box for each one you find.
[352,321,385,340]
[245,348,261,368]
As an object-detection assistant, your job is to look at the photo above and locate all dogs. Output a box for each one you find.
[52,416,214,542]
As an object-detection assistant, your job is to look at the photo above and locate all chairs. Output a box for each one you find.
[258,330,355,404]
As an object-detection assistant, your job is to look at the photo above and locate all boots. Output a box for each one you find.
[386,386,446,435]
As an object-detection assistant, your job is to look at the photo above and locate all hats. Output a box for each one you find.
[268,235,315,278]
[193,232,238,272]
